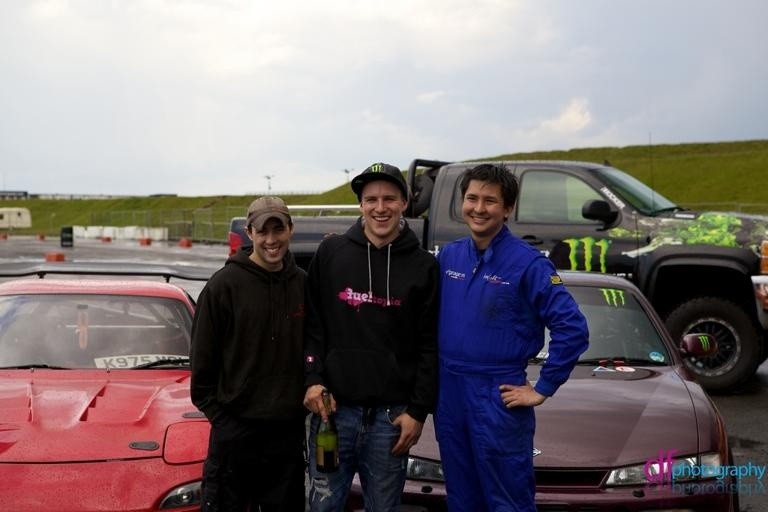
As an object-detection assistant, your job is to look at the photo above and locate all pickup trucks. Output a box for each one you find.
[226,158,768,397]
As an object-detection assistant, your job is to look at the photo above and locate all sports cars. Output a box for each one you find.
[0,265,230,511]
[347,271,741,512]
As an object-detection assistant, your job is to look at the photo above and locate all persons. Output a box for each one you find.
[185,195,310,509]
[299,161,444,509]
[433,163,591,509]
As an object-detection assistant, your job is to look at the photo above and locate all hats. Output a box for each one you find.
[351,163,408,200]
[247,197,290,230]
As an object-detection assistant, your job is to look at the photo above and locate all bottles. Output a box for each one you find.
[314,389,339,472]
